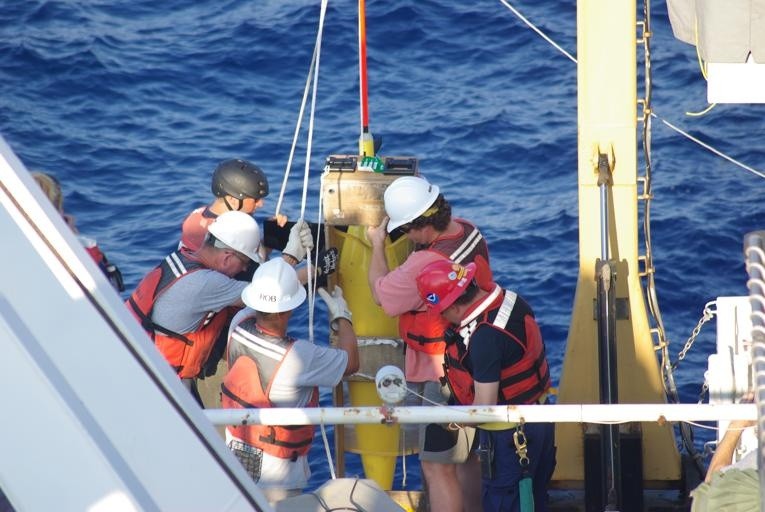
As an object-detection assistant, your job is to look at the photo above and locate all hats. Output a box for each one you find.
[240,256,308,314]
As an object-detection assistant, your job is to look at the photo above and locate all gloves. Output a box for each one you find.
[280,217,315,264]
[316,284,353,332]
[312,247,339,278]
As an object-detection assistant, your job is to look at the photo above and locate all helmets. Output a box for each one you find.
[383,174,441,235]
[207,210,264,264]
[415,259,478,320]
[211,158,270,200]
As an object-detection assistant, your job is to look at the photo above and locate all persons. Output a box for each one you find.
[688,389,763,512]
[365,173,493,511]
[219,216,363,505]
[178,158,287,264]
[125,211,339,401]
[31,168,124,300]
[414,254,558,511]
